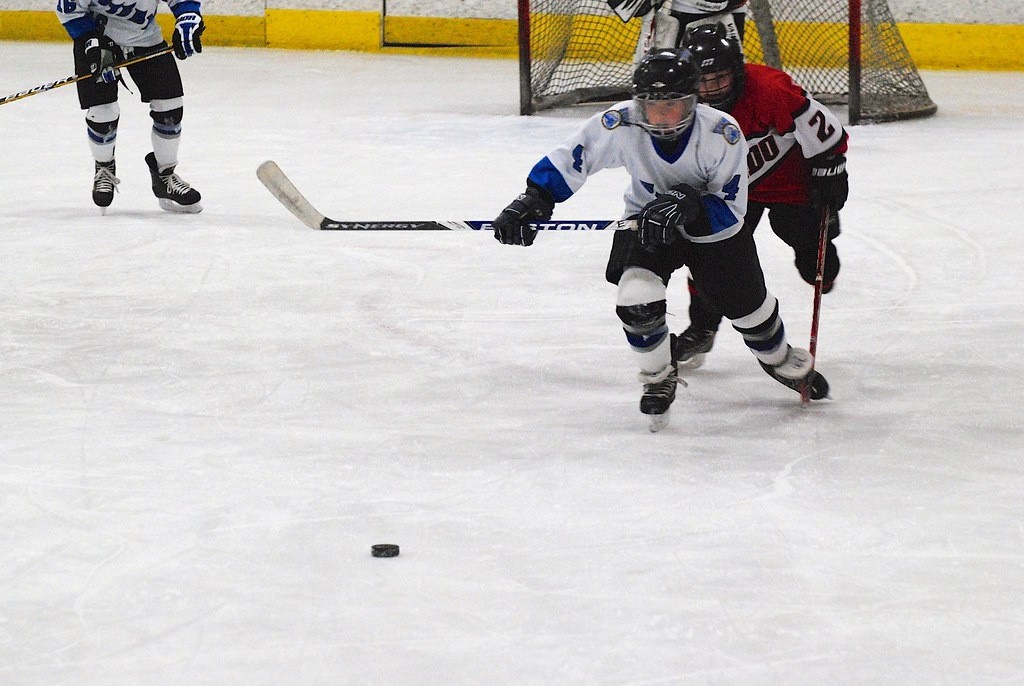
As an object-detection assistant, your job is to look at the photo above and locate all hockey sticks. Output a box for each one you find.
[257,161,639,232]
[800,204,831,405]
[0,45,176,105]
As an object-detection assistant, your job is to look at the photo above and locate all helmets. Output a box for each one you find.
[687,21,747,114]
[632,47,699,143]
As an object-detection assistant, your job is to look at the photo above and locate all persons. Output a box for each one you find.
[55,0,206,213]
[492,48,834,432]
[606,0,749,80]
[674,23,848,374]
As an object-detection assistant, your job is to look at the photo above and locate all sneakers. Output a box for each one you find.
[638,362,680,432]
[675,320,716,372]
[145,152,204,217]
[757,344,828,405]
[816,283,834,295]
[89,160,118,215]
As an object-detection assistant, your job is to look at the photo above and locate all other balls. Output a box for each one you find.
[371,544,400,558]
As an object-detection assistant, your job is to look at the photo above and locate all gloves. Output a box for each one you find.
[73,46,124,82]
[634,185,703,250]
[493,186,554,246]
[158,11,206,62]
